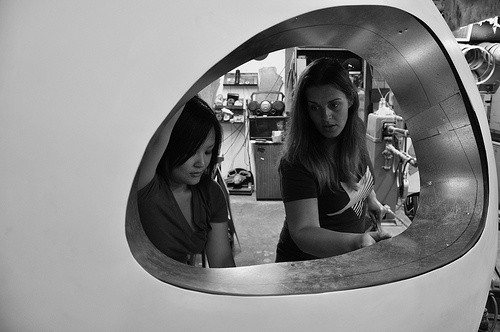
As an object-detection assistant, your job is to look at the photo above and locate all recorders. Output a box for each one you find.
[247,92,285,116]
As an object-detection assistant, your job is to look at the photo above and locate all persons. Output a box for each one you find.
[138,95,236,269]
[272,59,392,263]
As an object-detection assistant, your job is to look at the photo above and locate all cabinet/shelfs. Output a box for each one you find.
[218,83,257,124]
[245,90,290,198]
